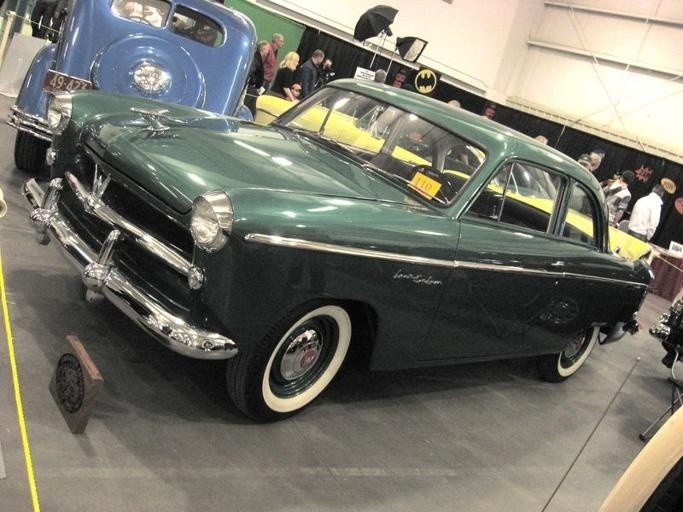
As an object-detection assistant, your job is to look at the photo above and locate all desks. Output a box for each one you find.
[646,240,683,303]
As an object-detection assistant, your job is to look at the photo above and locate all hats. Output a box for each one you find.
[580,153,593,162]
[291,83,302,90]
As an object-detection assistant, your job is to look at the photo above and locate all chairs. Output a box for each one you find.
[617,220,630,234]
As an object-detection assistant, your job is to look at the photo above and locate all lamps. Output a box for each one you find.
[382,37,429,84]
